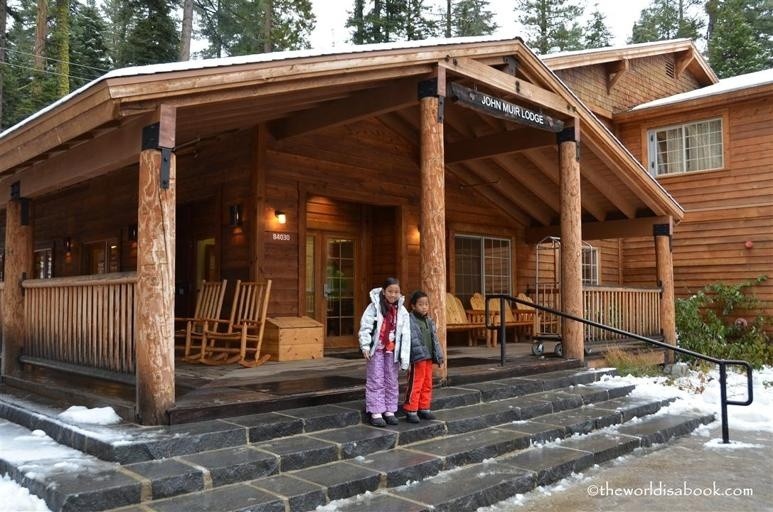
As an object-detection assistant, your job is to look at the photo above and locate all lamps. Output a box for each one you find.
[128,224,138,243]
[229,205,243,226]
[275,208,286,224]
[64,236,71,252]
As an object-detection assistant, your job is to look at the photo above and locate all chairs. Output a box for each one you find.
[197,279,272,368]
[175,280,229,364]
[446,291,543,347]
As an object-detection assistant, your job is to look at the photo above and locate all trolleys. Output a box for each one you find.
[522,232,602,358]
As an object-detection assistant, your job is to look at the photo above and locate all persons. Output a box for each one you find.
[401,291,446,423]
[358,277,411,427]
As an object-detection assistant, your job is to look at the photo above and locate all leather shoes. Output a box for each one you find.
[418,410,435,419]
[370,415,386,427]
[407,412,421,423]
[384,415,399,425]
